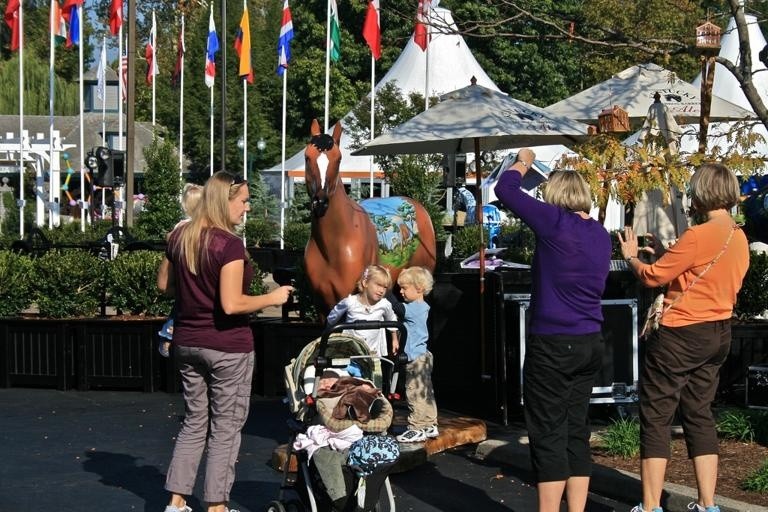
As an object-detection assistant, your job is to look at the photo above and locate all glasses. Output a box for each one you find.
[228,173,244,186]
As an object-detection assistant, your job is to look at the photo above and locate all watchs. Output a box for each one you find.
[626,254,637,264]
[519,158,529,170]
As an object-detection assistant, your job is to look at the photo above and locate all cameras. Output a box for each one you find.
[637,237,656,253]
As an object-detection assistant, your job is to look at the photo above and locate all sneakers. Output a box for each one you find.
[158,338,170,357]
[426,426,440,438]
[688,502,717,512]
[630,504,661,512]
[396,430,426,442]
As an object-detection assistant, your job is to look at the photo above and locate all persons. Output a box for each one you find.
[395,265,440,444]
[316,377,388,422]
[353,435,354,437]
[615,163,750,512]
[156,183,205,358]
[156,169,296,512]
[323,264,400,392]
[493,148,613,512]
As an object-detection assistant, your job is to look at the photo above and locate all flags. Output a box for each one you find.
[49,0,67,39]
[276,0,294,77]
[118,50,128,103]
[62,0,85,48]
[330,0,341,62]
[361,0,381,61]
[414,0,434,53]
[96,44,106,101]
[173,31,186,82]
[4,0,20,51]
[204,5,219,88]
[108,0,123,35]
[231,0,254,85]
[144,26,161,87]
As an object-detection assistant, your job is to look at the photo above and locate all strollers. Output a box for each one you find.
[265,320,408,512]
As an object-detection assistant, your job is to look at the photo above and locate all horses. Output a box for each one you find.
[302,118,435,333]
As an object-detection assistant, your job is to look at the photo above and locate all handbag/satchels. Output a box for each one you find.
[639,220,738,342]
